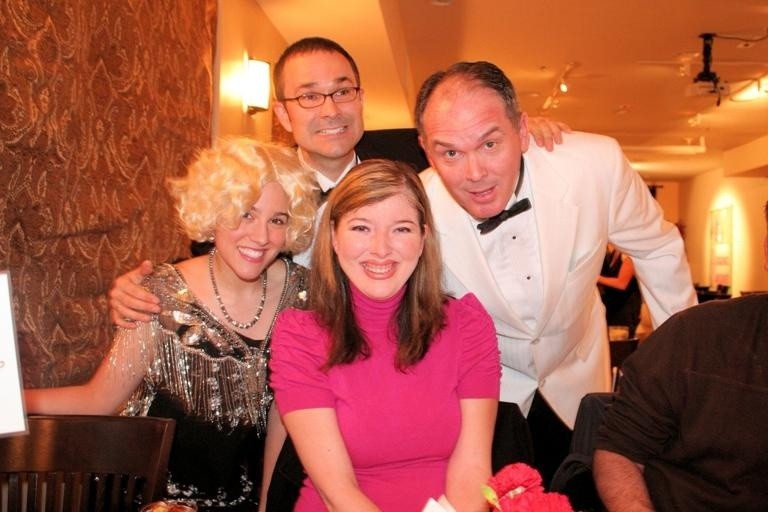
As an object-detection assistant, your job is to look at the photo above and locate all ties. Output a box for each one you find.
[477,198,531,235]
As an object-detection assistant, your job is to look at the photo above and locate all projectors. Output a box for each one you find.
[688,81,729,97]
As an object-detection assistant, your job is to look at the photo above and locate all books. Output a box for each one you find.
[0,270,30,439]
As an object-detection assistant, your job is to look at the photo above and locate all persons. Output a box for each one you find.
[22,34,768,512]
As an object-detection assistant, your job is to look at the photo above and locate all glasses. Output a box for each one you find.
[279,87,360,109]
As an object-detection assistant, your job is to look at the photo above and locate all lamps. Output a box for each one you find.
[238,58,271,116]
[678,30,768,106]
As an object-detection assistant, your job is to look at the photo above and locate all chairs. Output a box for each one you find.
[1,413,176,512]
